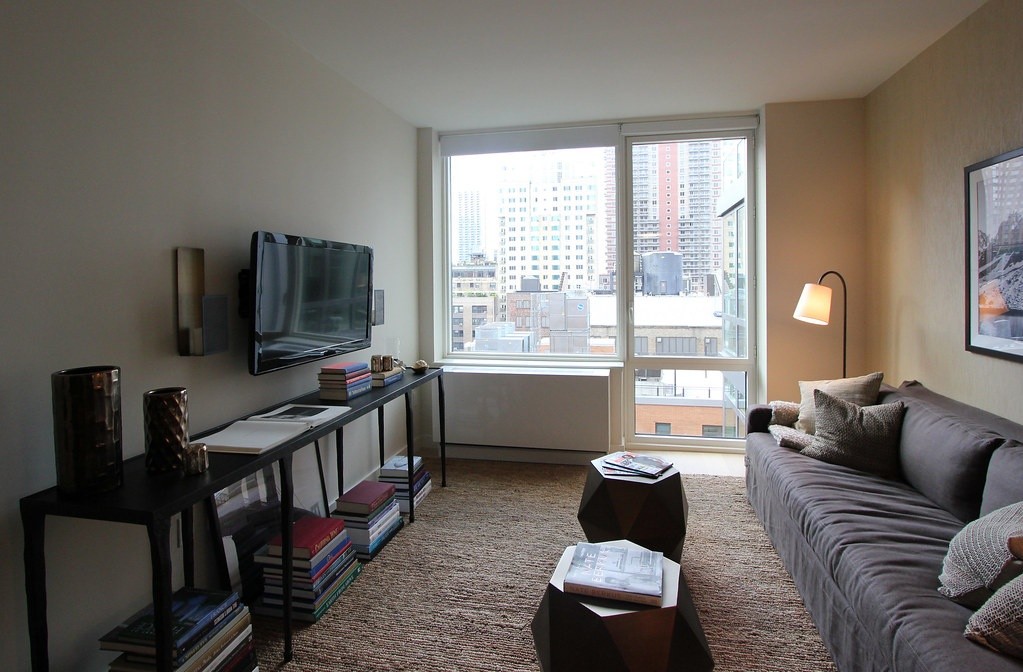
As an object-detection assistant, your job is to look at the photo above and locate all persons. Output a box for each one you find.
[622,455,665,469]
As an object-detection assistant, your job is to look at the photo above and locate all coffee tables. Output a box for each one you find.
[578,453,691,553]
[532,539,684,672]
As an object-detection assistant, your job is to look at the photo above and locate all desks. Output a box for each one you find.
[20,361,450,672]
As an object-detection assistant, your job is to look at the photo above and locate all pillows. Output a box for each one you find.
[798,388,906,478]
[961,572,1023,664]
[796,372,883,438]
[936,500,1023,612]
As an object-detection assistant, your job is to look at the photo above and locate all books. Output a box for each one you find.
[316,361,373,401]
[372,372,404,387]
[96,453,432,672]
[372,367,402,380]
[561,541,664,608]
[189,403,353,455]
[602,462,660,477]
[604,451,673,475]
[603,468,663,477]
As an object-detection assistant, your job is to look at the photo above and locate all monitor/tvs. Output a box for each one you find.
[249,231,372,376]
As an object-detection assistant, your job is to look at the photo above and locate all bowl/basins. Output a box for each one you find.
[411,366,430,374]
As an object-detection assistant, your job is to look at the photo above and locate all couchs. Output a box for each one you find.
[740,378,1023,671]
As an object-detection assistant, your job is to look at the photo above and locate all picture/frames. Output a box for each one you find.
[964,148,1023,363]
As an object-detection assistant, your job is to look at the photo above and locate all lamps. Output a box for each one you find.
[791,269,849,380]
